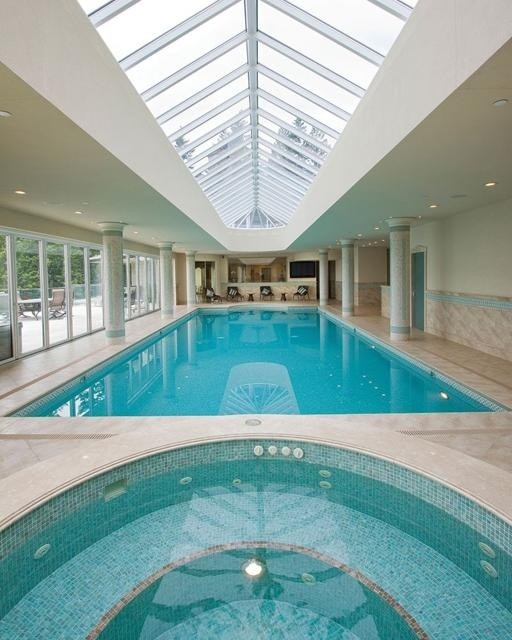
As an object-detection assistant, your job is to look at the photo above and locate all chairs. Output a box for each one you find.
[204,284,310,304]
[0,287,66,321]
